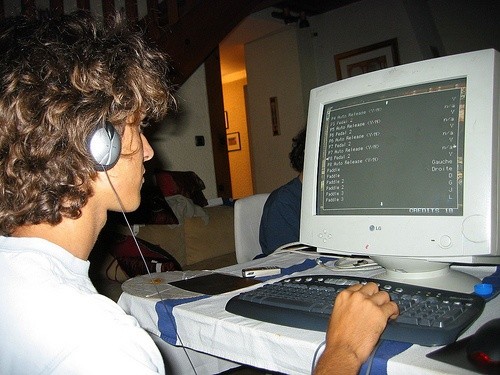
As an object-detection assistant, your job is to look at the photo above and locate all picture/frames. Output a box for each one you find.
[227,132,241,151]
[333,38,399,81]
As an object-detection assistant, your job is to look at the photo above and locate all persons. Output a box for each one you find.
[0,8,399,375]
[259,124,307,256]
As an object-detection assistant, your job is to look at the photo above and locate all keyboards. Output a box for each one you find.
[226,274,485,347]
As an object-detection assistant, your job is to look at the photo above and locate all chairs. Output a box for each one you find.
[234,192,273,262]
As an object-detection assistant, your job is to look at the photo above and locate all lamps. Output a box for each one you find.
[271,6,310,29]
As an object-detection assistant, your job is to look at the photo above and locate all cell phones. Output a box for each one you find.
[242,265,281,277]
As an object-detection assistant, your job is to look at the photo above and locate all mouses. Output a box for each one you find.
[465,319,500,371]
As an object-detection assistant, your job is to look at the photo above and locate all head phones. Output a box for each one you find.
[86,123,122,171]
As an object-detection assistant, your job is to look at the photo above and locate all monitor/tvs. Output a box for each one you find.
[299,49,500,292]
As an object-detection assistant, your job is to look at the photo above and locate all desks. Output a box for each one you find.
[117,248,500,375]
